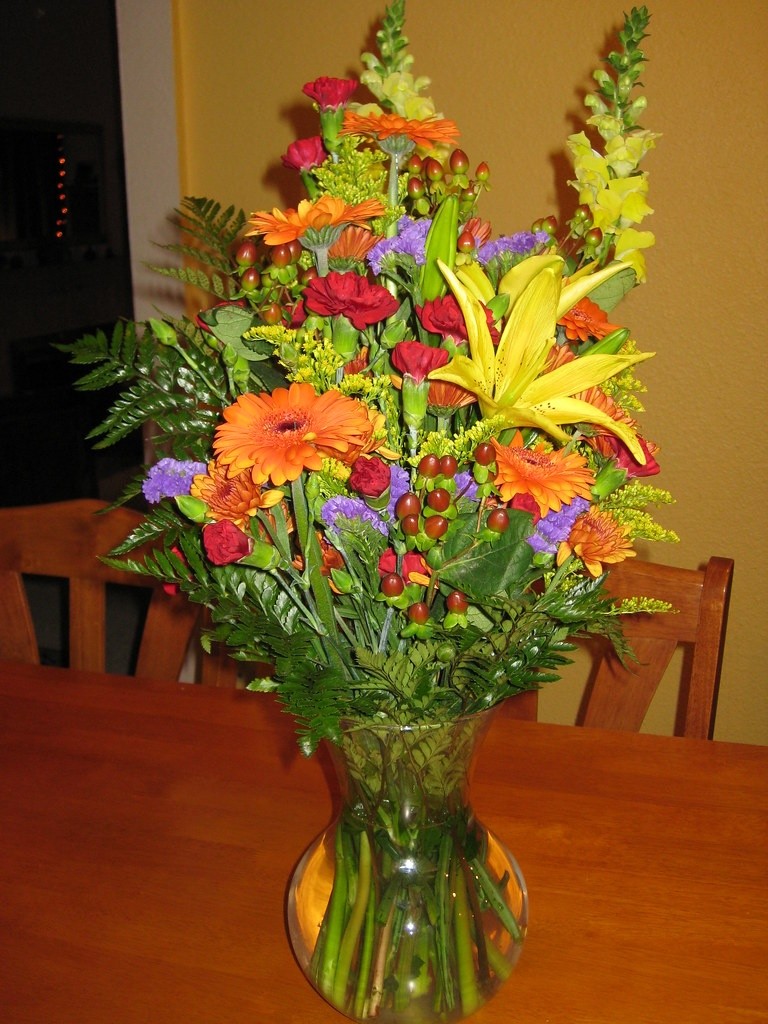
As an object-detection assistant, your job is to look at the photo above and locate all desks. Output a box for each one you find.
[0,653,768,1024]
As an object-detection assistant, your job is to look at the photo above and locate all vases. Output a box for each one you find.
[283,704,527,1024]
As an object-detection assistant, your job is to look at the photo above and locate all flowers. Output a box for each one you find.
[45,0,662,750]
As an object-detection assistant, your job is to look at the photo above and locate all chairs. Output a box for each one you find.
[490,497,736,742]
[0,497,286,696]
